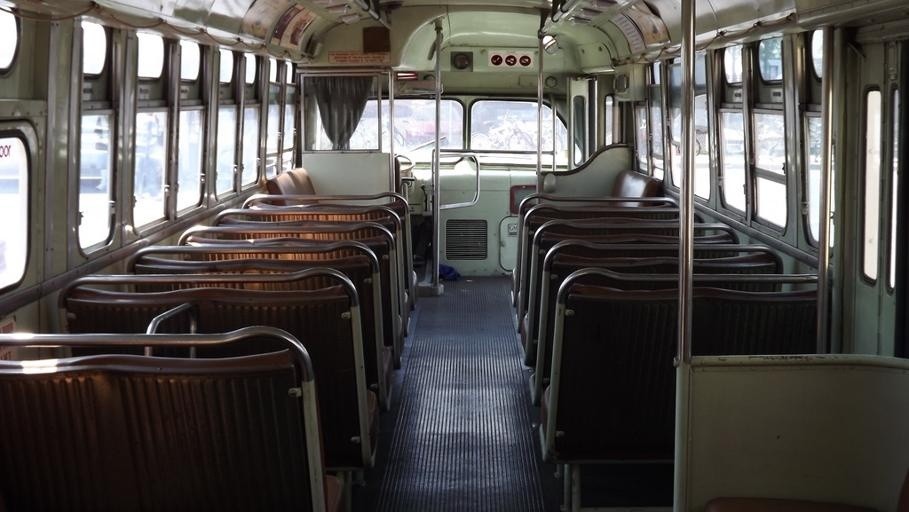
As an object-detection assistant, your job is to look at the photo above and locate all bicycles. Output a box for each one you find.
[471,115,534,150]
